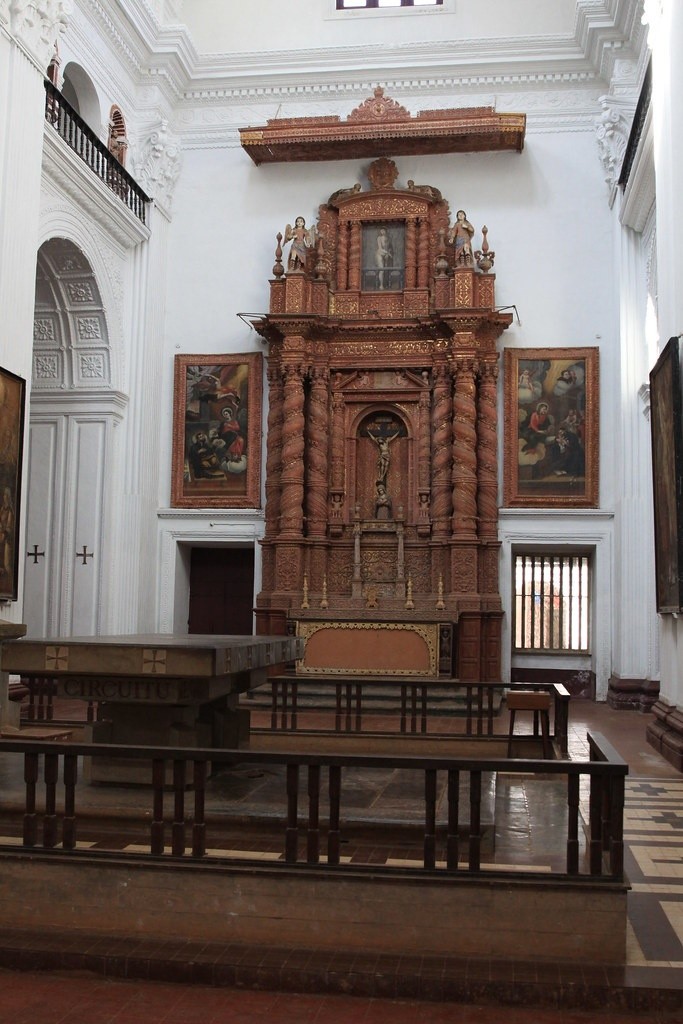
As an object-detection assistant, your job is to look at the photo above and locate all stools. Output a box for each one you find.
[504,689,551,760]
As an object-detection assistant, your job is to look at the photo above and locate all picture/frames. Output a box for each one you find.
[172,351,267,508]
[504,346,600,508]
[0,364,27,601]
[649,334,683,617]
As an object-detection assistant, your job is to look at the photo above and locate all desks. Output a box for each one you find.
[6,635,304,785]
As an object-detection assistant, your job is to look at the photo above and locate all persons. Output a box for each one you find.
[375,484,393,519]
[415,490,430,517]
[288,217,311,273]
[335,183,361,200]
[408,180,438,199]
[330,493,343,518]
[367,428,401,480]
[108,130,121,180]
[448,210,474,268]
